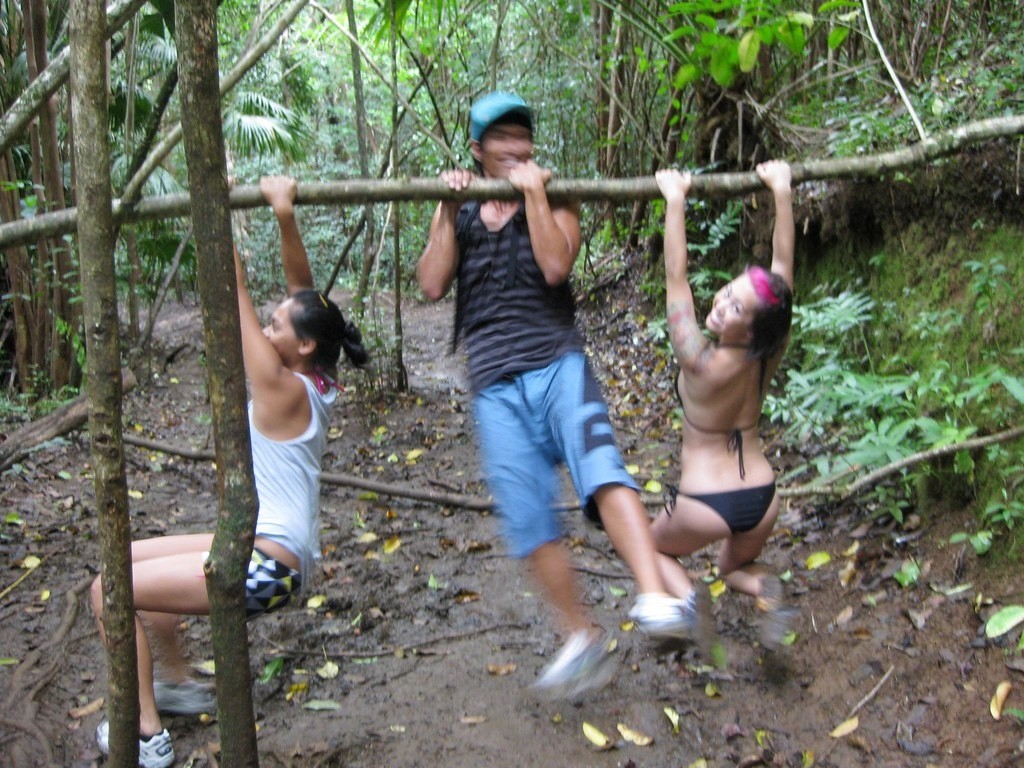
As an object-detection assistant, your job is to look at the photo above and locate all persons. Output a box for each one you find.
[414,90,687,700]
[650,157,796,651]
[93,172,371,768]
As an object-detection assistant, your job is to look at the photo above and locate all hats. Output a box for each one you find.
[471,93,533,141]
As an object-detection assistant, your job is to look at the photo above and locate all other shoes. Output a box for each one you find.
[539,630,610,689]
[628,595,689,639]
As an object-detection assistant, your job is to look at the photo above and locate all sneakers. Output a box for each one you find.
[96,719,175,768]
[152,680,214,714]
